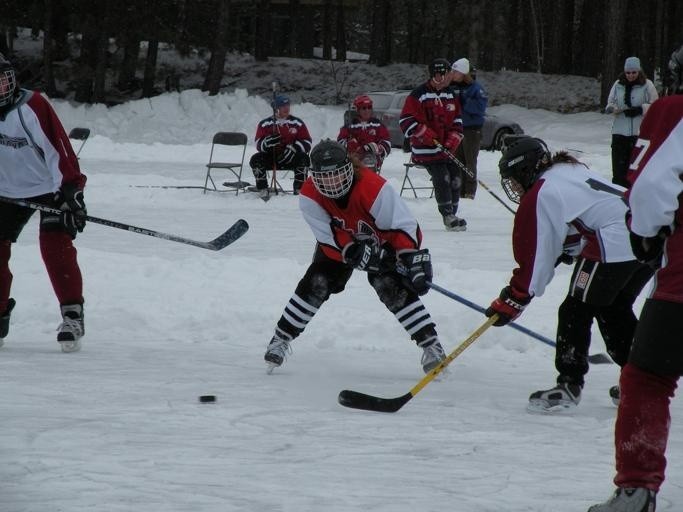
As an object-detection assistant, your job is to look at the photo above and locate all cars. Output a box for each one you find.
[357,87,526,154]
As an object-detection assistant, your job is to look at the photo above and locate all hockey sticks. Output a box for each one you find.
[426,282,614,364]
[0,197,249,251]
[339,312,499,412]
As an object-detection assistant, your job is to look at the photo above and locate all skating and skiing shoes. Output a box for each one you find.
[527,379,582,405]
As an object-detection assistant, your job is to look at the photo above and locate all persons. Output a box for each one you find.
[585,95,683,512]
[451,56,489,200]
[264,140,448,372]
[605,56,660,187]
[398,58,467,228]
[247,95,312,195]
[336,95,392,174]
[0,54,87,341]
[485,137,659,403]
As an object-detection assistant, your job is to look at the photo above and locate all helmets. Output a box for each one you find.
[309,138,354,200]
[497,135,552,206]
[352,95,373,113]
[428,58,451,80]
[270,96,291,110]
[0,53,17,109]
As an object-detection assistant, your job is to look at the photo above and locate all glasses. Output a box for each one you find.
[362,104,373,110]
[625,70,637,75]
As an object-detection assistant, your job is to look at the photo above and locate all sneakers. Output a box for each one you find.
[0,298,17,338]
[586,486,657,511]
[609,384,620,400]
[441,210,467,227]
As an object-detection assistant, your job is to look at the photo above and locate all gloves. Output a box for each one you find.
[344,242,393,273]
[623,105,643,120]
[414,124,440,147]
[440,129,466,161]
[256,133,283,153]
[484,283,536,327]
[55,177,88,238]
[398,248,434,296]
[275,142,305,168]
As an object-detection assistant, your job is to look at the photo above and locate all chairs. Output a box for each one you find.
[399,153,434,199]
[266,168,297,195]
[205,132,247,196]
[66,128,90,160]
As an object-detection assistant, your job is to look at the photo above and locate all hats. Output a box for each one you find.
[624,56,641,73]
[451,56,471,75]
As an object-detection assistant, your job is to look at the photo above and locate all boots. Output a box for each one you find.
[264,325,294,366]
[418,334,447,377]
[55,300,86,342]
